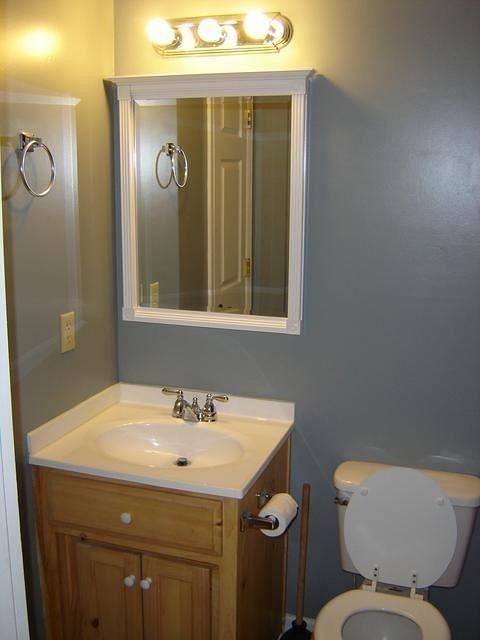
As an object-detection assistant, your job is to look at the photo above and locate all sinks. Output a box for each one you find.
[100,417,242,470]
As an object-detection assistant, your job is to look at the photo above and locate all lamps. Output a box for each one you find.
[145,12,293,57]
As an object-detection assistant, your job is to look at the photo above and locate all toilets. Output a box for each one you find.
[315,457,479,638]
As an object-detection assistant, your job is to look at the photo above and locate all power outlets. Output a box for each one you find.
[149,281,160,308]
[61,311,76,353]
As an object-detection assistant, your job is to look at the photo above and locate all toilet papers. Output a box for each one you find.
[260,492,299,541]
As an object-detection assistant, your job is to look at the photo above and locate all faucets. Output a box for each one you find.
[171,396,201,424]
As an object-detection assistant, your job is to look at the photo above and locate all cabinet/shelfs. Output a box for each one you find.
[31,435,291,640]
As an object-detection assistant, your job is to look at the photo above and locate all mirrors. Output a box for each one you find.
[103,68,315,336]
